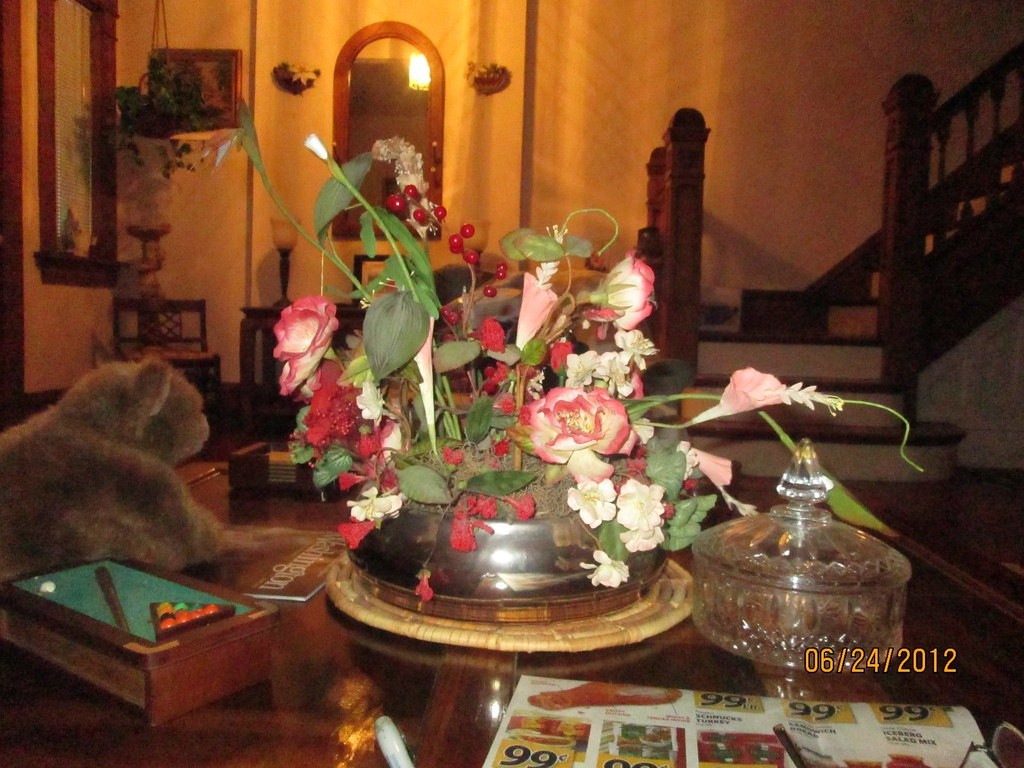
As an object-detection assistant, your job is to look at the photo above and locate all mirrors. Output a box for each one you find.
[331,20,445,242]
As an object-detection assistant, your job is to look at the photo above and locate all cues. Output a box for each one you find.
[94,566,130,633]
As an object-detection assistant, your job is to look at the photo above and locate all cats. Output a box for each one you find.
[0,355,228,586]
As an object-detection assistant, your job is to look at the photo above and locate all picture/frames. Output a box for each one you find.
[353,254,392,306]
[153,48,242,128]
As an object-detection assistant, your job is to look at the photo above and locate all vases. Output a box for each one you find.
[475,80,509,94]
[347,470,660,604]
[274,69,314,95]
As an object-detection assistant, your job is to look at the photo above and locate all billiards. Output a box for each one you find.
[157,602,220,629]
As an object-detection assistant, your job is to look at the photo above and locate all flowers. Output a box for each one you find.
[235,104,844,590]
[465,61,510,81]
[280,62,319,85]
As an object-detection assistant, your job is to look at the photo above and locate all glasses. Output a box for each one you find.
[960,722,1024,768]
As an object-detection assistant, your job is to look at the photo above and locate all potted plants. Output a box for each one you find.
[114,53,218,177]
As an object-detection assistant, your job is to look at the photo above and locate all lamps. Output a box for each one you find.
[461,218,489,273]
[270,217,300,308]
[409,51,431,92]
